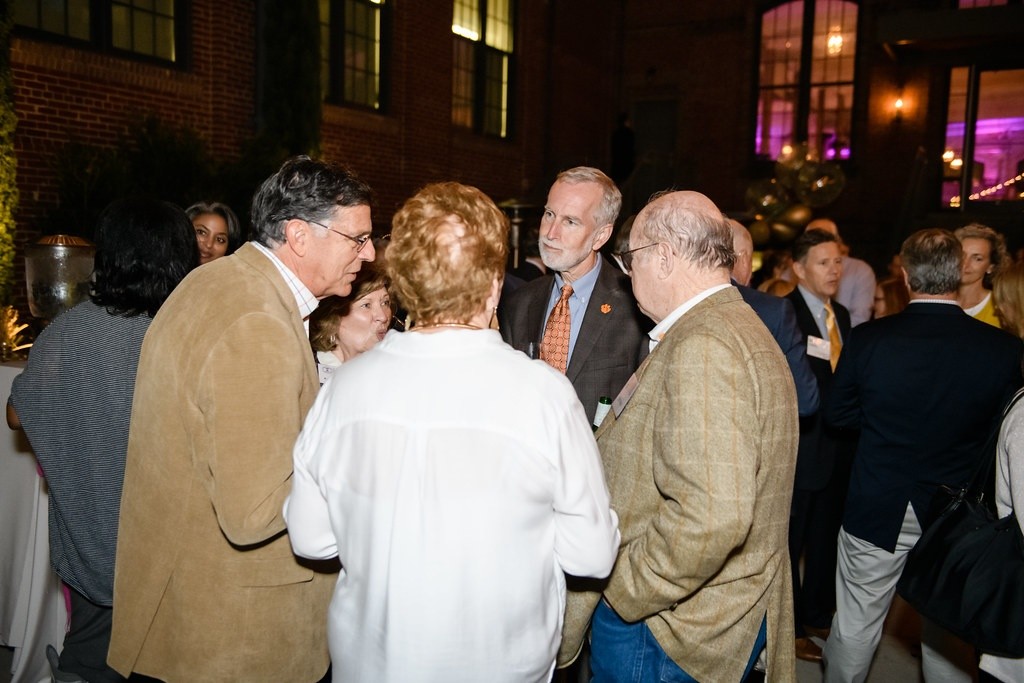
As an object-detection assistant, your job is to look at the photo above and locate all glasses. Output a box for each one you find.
[620,242,676,269]
[286,215,371,253]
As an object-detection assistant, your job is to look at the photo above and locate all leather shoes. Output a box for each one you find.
[809,626,830,640]
[793,636,825,661]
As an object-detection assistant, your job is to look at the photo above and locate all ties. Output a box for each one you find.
[824,303,843,373]
[539,285,574,375]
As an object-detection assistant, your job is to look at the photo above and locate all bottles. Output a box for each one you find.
[591,396,612,433]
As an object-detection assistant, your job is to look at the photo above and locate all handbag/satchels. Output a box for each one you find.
[896,390,1024,660]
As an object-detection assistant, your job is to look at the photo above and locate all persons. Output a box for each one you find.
[6,154,1024,683]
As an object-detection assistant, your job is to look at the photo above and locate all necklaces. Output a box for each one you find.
[409,323,482,331]
